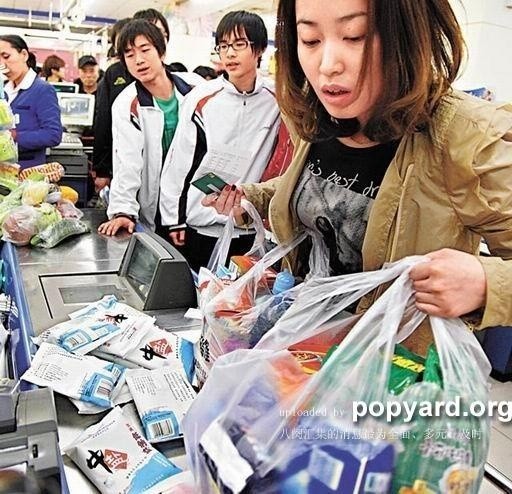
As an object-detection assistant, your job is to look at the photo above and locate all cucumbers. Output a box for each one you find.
[30,218,88,248]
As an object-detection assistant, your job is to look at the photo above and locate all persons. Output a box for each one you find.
[0,1,512,359]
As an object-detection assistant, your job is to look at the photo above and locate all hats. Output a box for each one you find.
[78,55,96,68]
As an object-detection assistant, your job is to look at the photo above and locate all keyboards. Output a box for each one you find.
[60,134,81,143]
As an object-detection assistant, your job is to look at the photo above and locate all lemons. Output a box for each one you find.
[61,186,80,204]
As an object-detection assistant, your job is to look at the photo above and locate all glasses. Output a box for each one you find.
[214,40,254,53]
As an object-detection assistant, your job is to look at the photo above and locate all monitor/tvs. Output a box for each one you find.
[58,94,95,132]
[52,82,79,96]
